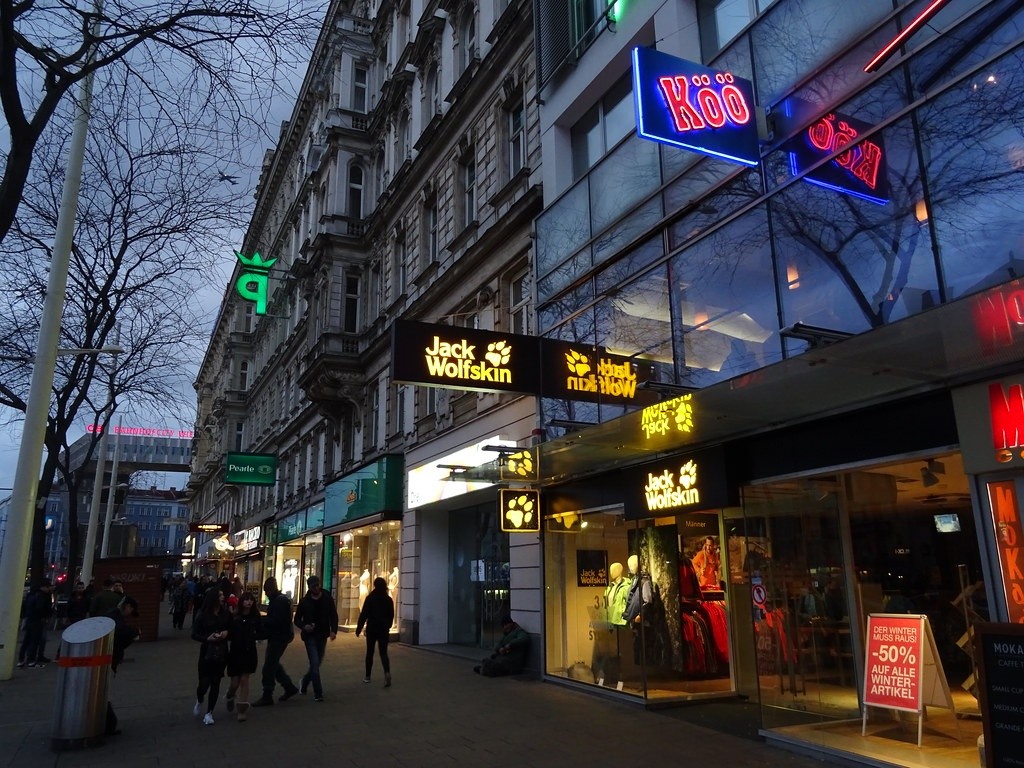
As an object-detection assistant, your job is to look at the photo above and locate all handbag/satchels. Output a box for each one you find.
[168,605,175,614]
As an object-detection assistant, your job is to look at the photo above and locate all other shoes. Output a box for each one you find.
[279,688,299,701]
[384,677,391,687]
[363,677,370,682]
[28,662,36,669]
[16,662,25,669]
[35,664,46,669]
[300,679,308,695]
[250,698,273,707]
[315,695,323,700]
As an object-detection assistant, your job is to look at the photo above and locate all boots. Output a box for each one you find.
[237,702,250,720]
[223,690,237,712]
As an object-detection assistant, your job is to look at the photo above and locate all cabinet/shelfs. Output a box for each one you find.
[792,627,853,688]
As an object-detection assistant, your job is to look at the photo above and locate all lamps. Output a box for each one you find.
[580,520,588,528]
[613,514,624,527]
[810,482,828,501]
[920,459,939,487]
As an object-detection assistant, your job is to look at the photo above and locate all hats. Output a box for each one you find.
[308,576,320,587]
[39,578,55,588]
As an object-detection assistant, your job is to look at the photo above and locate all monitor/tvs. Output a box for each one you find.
[932,513,963,535]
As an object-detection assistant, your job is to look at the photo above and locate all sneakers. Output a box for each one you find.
[203,713,214,724]
[194,700,203,716]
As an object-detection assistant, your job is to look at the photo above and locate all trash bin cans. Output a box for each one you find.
[49,616,116,749]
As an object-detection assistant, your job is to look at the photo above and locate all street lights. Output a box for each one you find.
[101,415,127,559]
[79,322,128,589]
[0,0,125,680]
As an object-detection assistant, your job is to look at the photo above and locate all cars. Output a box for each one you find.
[24,566,80,588]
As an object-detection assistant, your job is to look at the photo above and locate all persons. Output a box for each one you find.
[689,536,722,593]
[251,577,299,706]
[604,555,659,670]
[191,588,236,723]
[223,593,262,721]
[473,616,532,677]
[17,577,141,676]
[356,577,394,687]
[819,573,849,623]
[294,576,338,701]
[159,571,243,631]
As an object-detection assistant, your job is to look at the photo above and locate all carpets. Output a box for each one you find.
[651,698,839,743]
